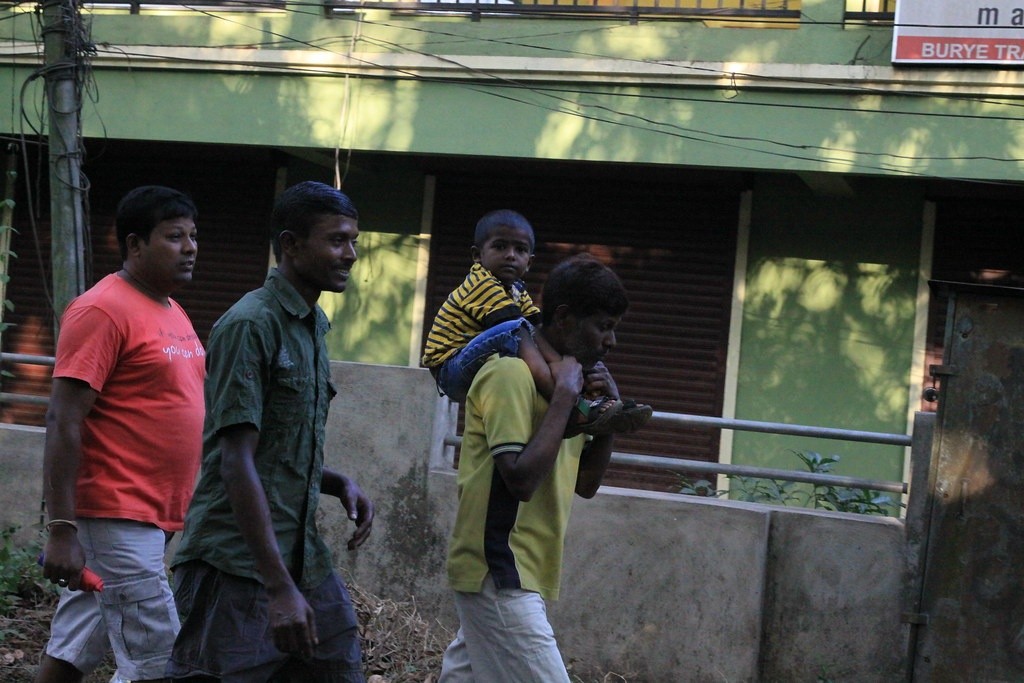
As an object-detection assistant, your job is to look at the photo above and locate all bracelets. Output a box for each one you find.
[46,519,79,533]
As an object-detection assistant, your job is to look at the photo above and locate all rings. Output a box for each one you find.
[59,577,68,582]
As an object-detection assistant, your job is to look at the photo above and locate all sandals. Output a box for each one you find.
[562,395,622,439]
[584,399,653,435]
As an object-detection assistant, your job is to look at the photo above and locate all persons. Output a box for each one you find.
[162,183,372,683]
[420,209,654,437]
[437,256,619,683]
[34,185,206,683]
